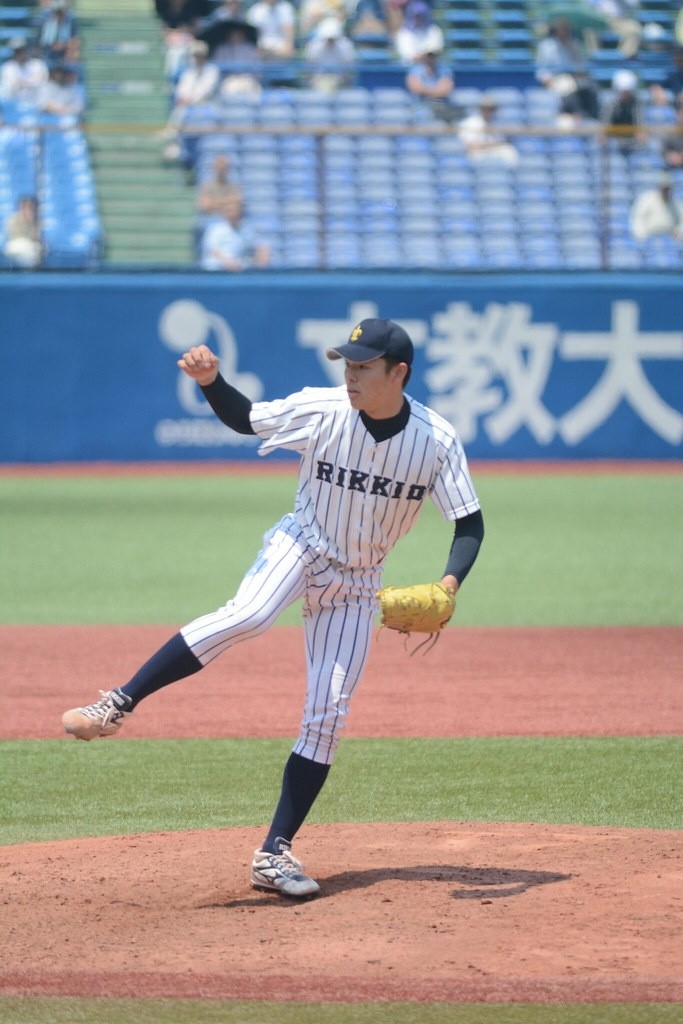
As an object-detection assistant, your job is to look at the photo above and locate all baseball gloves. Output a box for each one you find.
[374,582,456,634]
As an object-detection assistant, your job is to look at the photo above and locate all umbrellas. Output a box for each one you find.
[194,19,260,49]
[542,9,608,29]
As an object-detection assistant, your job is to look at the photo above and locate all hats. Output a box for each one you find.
[326,318,414,366]
[0,0,683,190]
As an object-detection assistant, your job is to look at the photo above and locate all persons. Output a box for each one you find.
[5,196,43,269]
[628,174,683,240]
[155,0,462,160]
[0,0,85,116]
[60,318,485,899]
[527,1,683,167]
[193,159,268,271]
[458,98,517,160]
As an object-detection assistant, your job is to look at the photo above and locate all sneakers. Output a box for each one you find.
[249,836,321,900]
[61,686,134,740]
